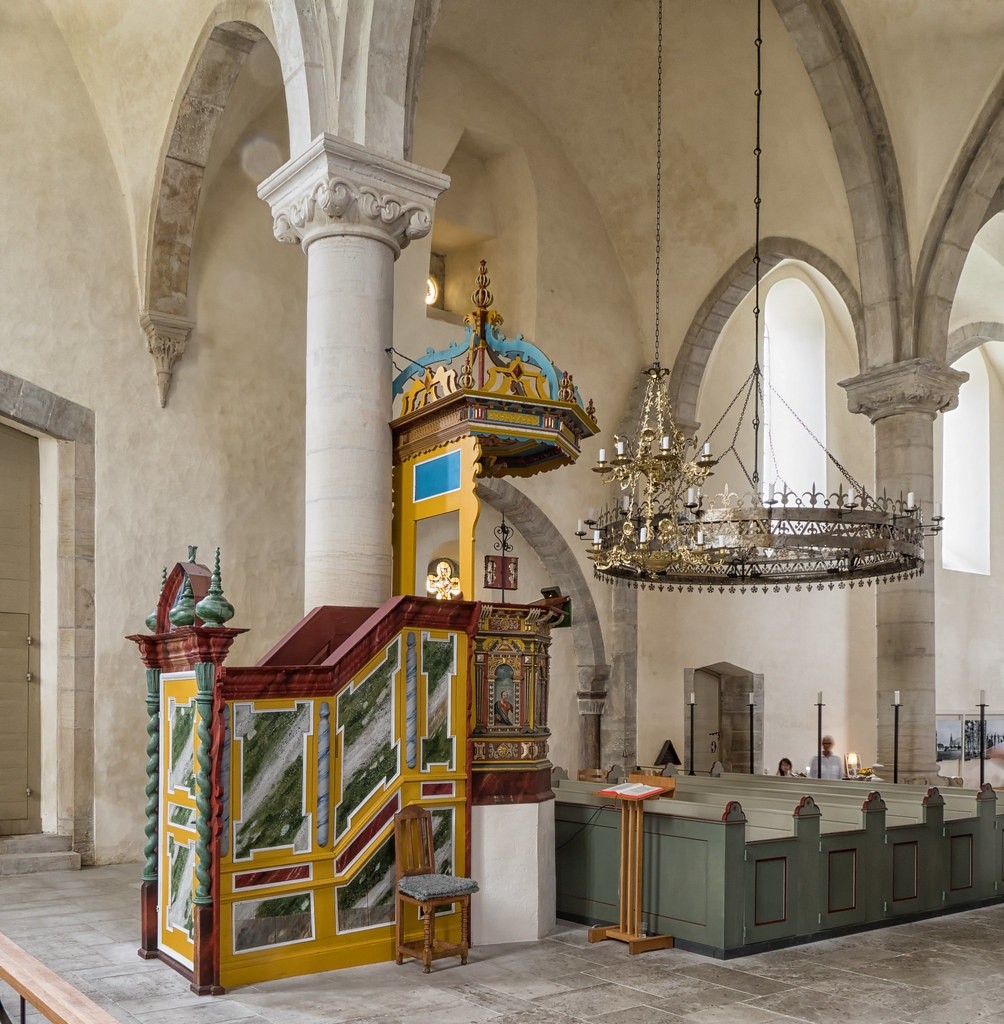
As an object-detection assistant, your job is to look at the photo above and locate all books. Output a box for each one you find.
[600,783,664,799]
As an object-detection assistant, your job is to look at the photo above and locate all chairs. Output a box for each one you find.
[392,804,478,974]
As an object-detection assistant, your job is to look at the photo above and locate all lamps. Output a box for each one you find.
[575,0,945,594]
[847,753,858,778]
[593,0,717,491]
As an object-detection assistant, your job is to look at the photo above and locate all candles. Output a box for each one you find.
[691,692,695,703]
[895,690,900,704]
[818,690,822,704]
[980,689,986,704]
[750,692,754,704]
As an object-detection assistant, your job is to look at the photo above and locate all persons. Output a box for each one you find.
[777,758,801,777]
[810,736,842,780]
